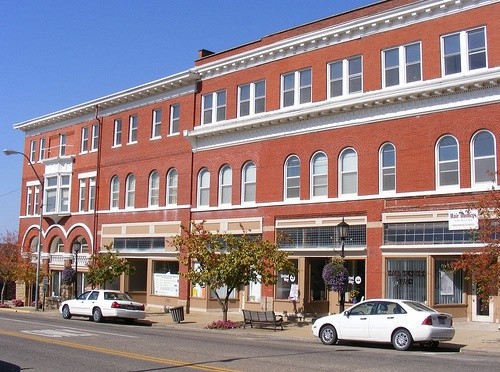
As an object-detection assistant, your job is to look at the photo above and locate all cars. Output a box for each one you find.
[311,298,456,351]
[58,289,146,323]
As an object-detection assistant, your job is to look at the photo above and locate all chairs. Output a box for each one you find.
[393,308,401,314]
[380,305,388,314]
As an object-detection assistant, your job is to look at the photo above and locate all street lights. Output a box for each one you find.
[72,238,81,299]
[336,217,351,313]
[3,148,45,311]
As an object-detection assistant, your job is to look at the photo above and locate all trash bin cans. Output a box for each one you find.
[169,305,184,322]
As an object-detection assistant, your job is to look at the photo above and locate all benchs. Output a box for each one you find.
[242,309,284,332]
